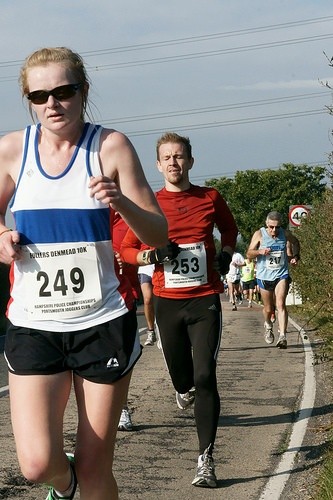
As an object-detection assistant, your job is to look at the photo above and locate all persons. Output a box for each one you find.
[246,211,298,349]
[0,48,168,500]
[112,210,141,431]
[224,252,257,311]
[120,131,239,487]
[138,264,157,345]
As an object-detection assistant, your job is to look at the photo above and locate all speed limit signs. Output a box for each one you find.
[289,205,314,231]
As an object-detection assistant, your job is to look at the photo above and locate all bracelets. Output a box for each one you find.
[0,230,11,235]
[288,256,293,262]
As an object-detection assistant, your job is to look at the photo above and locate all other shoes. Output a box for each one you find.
[232,305,237,311]
[239,301,242,305]
[270,311,276,322]
[248,303,252,307]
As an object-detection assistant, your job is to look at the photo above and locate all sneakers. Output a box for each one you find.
[45,453,78,500]
[276,338,287,349]
[176,386,195,410]
[263,321,274,344]
[191,443,217,487]
[117,409,133,431]
[144,330,156,345]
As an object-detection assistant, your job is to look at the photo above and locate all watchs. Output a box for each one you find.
[141,250,151,264]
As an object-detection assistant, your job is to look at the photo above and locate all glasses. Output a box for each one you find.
[27,82,84,105]
[268,224,281,229]
